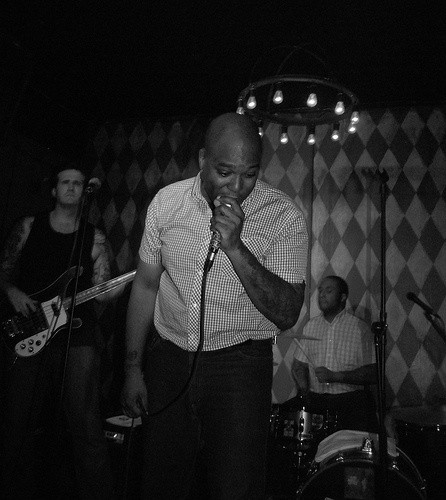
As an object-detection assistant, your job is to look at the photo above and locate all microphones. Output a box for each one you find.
[407,292,439,318]
[203,198,232,271]
[85,178,101,194]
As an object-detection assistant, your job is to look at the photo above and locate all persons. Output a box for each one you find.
[291,276,379,430]
[118,112,308,500]
[0,163,127,500]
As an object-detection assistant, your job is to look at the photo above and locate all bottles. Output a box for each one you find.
[297,390,312,444]
[271,405,279,436]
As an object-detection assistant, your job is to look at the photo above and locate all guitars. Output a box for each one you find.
[0,264,137,359]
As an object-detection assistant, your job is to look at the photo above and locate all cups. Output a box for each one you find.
[283,420,294,436]
[326,409,337,428]
[312,414,323,430]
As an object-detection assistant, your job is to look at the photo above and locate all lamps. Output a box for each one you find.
[235,44,360,145]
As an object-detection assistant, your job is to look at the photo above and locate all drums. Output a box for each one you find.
[272,407,343,471]
[297,428,426,500]
